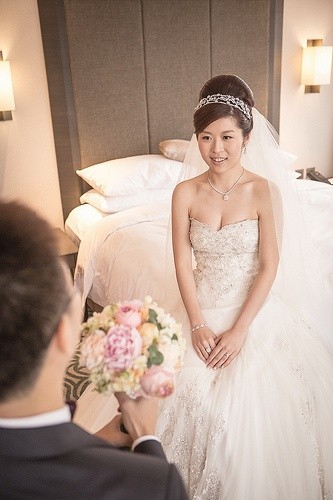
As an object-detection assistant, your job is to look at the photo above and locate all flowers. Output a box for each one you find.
[75,295,183,397]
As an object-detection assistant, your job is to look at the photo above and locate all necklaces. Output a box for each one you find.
[207,166,245,200]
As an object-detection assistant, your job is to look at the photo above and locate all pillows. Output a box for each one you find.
[76,137,196,214]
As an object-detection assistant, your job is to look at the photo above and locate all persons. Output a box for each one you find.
[73,74,333,500]
[0,201,189,500]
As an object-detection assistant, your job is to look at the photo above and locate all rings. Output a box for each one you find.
[225,354,230,357]
[206,348,211,353]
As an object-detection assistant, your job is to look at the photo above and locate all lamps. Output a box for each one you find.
[300,38,333,93]
[0,51,17,121]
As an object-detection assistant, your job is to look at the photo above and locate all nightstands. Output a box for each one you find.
[47,228,80,269]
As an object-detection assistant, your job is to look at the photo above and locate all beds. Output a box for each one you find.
[63,176,333,500]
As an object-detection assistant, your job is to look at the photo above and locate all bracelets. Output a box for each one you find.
[190,324,208,333]
